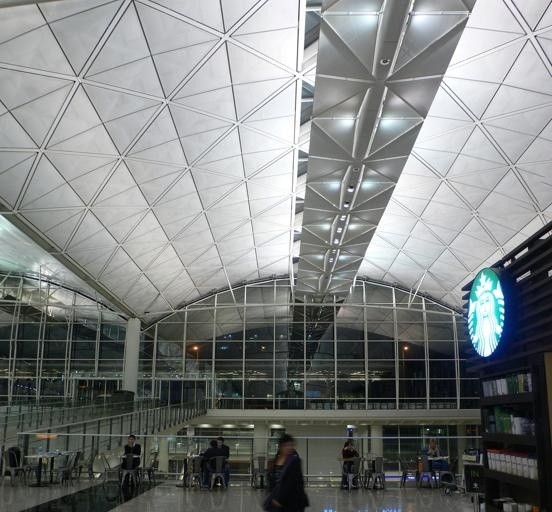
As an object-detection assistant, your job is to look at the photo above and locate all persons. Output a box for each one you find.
[262,434,311,512]
[198,436,230,488]
[421,438,448,469]
[120,435,141,486]
[339,441,361,489]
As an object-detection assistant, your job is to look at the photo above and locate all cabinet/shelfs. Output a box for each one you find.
[468,351,550,511]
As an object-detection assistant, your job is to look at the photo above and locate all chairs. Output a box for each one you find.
[119,452,155,490]
[399,453,459,493]
[2,450,97,489]
[184,454,226,490]
[338,458,384,490]
[251,454,273,488]
